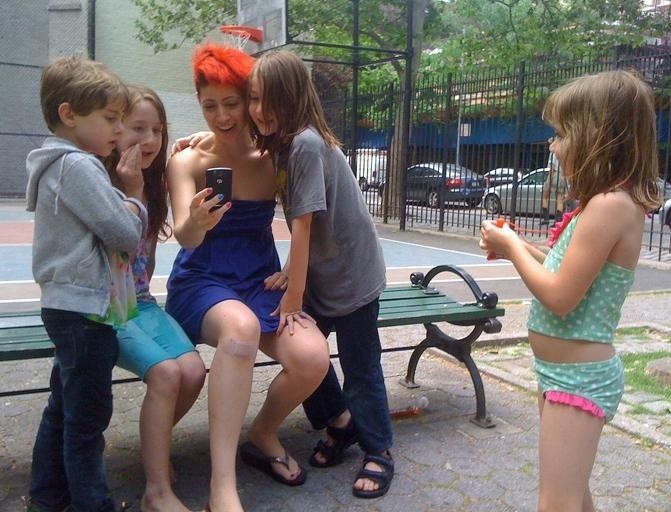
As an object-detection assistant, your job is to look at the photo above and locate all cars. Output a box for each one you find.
[658,177,671,227]
[345,148,579,218]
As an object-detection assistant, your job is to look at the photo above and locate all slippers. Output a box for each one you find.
[244,442,307,486]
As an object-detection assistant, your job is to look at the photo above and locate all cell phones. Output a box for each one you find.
[206,168,232,209]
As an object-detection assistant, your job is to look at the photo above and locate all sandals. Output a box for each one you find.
[353,449,394,497]
[309,417,360,468]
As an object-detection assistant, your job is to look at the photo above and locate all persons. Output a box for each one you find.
[171,51,395,498]
[164,42,330,511]
[540,153,567,228]
[95,83,207,512]
[24,50,149,510]
[477,65,665,511]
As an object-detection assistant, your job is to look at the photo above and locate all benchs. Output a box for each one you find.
[1,266,506,428]
